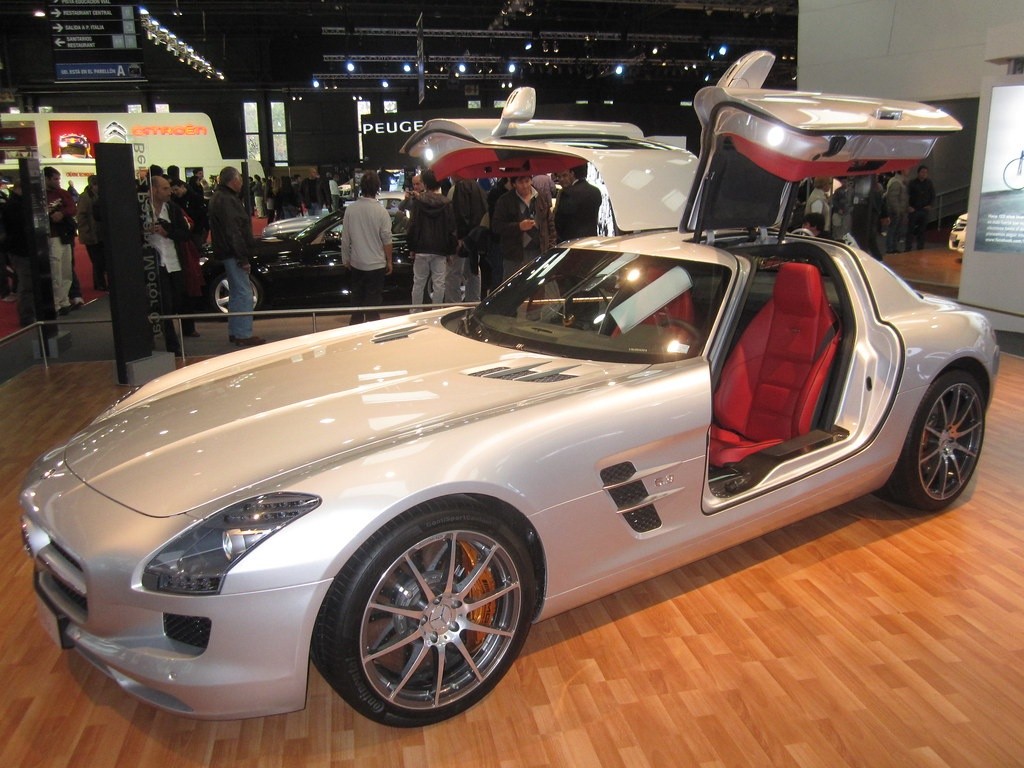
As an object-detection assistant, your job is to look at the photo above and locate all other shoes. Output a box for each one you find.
[174,350,187,357]
[58,307,70,315]
[2,292,18,302]
[73,297,83,305]
[191,332,200,337]
[229,334,266,347]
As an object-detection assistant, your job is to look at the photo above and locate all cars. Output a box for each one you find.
[191,189,483,320]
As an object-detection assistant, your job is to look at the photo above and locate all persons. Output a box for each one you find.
[801,164,935,262]
[0,156,602,349]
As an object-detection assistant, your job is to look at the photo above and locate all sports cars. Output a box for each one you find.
[20,49,999,729]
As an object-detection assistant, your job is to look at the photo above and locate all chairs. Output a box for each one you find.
[615,270,696,352]
[709,264,841,469]
